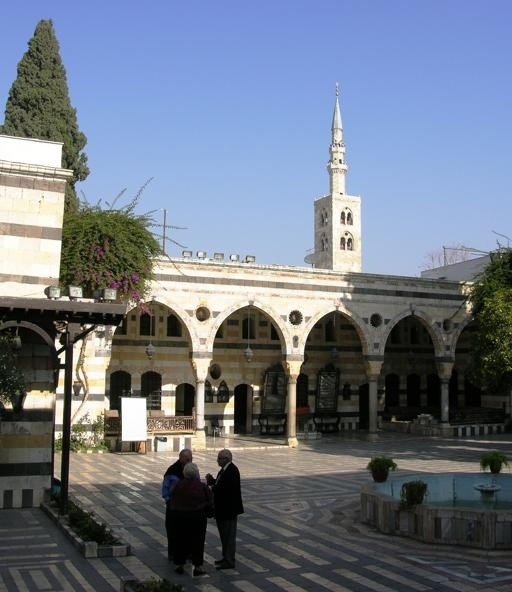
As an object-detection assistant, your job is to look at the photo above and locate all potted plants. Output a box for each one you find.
[366,453,397,482]
[480,450,512,474]
[399,480,427,508]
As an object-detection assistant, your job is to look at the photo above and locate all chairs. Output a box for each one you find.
[210,417,224,438]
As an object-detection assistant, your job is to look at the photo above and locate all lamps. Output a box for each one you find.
[331,312,339,361]
[244,307,254,362]
[12,326,23,351]
[145,306,155,360]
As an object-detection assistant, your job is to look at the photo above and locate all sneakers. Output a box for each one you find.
[194,568,205,576]
[217,563,234,570]
[176,568,185,575]
[215,559,223,565]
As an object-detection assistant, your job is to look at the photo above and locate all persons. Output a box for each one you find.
[205,448,244,570]
[167,462,213,576]
[162,448,201,561]
